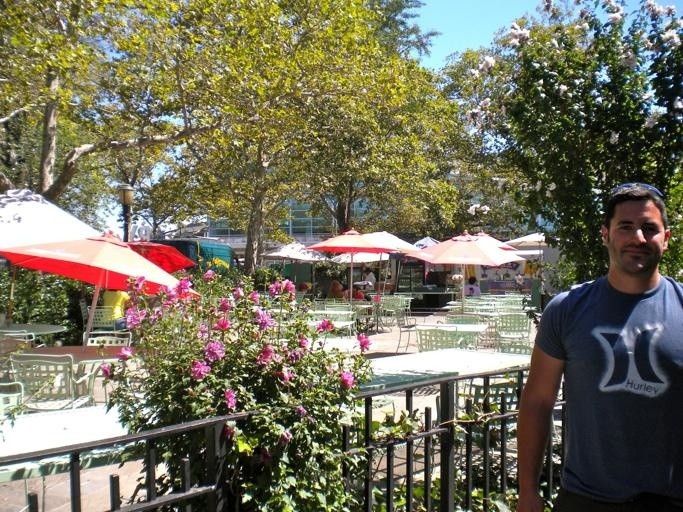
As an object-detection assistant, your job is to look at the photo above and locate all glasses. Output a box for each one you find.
[610,182,664,197]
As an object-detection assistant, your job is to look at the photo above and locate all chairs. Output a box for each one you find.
[0,291,369,411]
[369,291,534,356]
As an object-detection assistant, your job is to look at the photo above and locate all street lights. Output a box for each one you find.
[117,182,133,241]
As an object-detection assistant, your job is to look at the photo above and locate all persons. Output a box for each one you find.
[59,288,86,346]
[516,182,683,511]
[100,288,132,330]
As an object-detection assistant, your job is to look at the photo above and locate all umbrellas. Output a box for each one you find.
[0,229,202,347]
[0,186,98,249]
[123,238,200,276]
